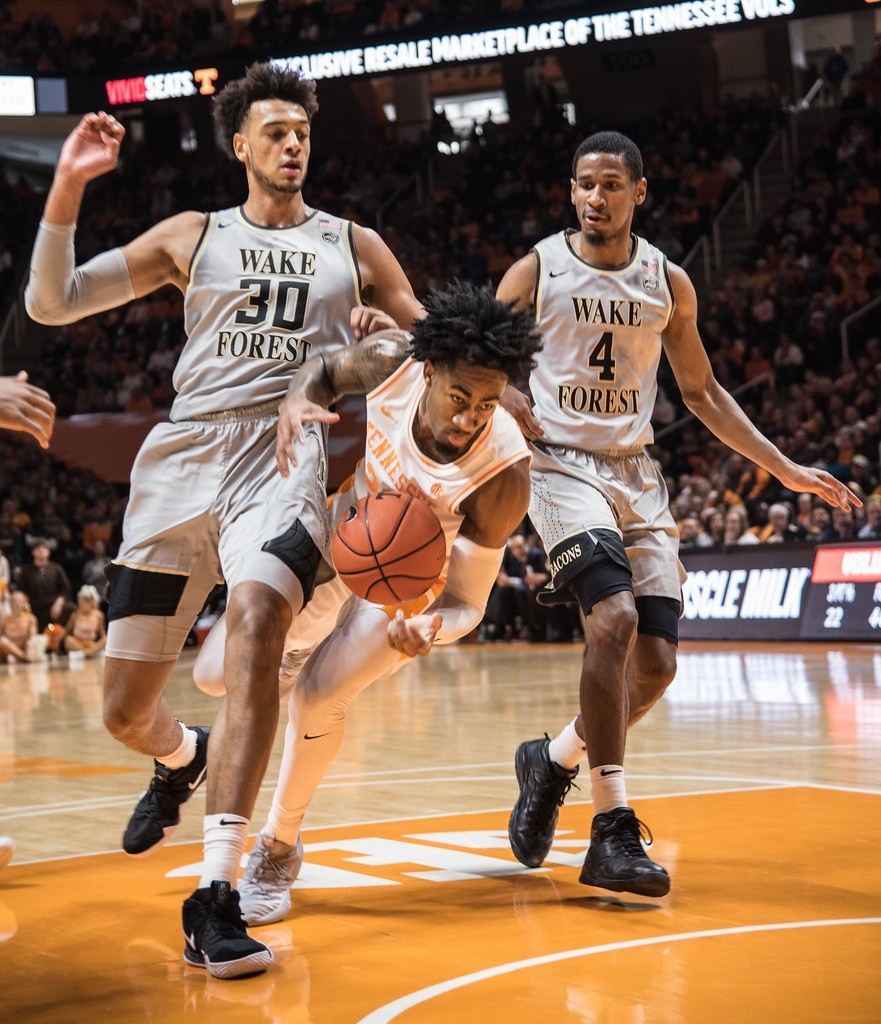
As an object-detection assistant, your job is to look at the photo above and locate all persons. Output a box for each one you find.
[193,281,544,928]
[0,1,881,664]
[24,67,430,979]
[494,132,863,898]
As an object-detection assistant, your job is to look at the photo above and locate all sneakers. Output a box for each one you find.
[577,806,672,899]
[278,648,314,696]
[180,881,274,979]
[237,830,304,926]
[507,731,581,868]
[121,726,220,860]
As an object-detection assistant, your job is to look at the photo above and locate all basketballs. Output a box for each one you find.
[329,489,447,605]
